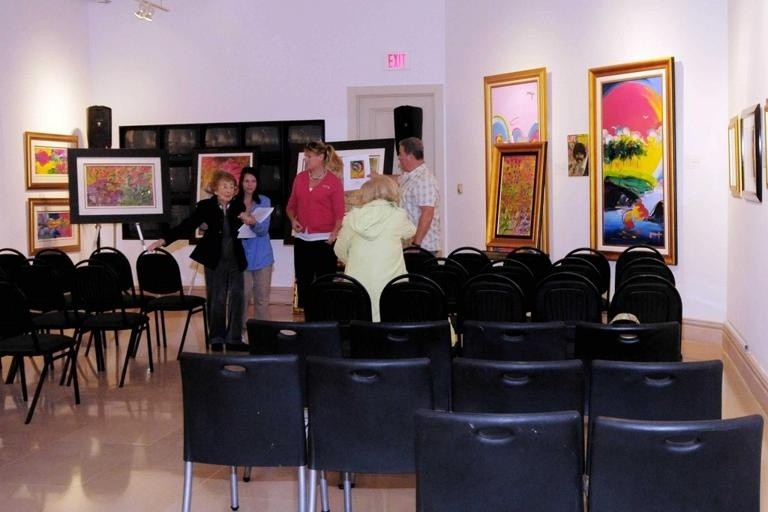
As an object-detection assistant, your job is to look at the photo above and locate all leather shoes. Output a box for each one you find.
[210,343,251,353]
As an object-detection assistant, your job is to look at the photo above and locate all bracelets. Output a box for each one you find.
[153,241,162,247]
[412,241,420,248]
[330,232,337,236]
[288,215,295,219]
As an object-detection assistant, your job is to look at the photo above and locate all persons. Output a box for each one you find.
[235,166,274,339]
[148,170,251,352]
[569,142,588,175]
[368,137,442,257]
[287,141,345,300]
[333,174,416,323]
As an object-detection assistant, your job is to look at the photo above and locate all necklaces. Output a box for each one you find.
[309,172,326,180]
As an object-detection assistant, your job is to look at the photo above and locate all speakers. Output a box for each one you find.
[394,106,423,156]
[88,106,111,148]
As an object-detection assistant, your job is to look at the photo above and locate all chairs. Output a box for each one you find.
[1,242,216,426]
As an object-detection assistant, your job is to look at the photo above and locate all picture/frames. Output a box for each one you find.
[187,144,263,243]
[482,142,547,253]
[483,65,552,260]
[26,196,84,256]
[281,137,398,244]
[63,144,174,226]
[22,129,80,192]
[725,99,768,205]
[587,54,680,267]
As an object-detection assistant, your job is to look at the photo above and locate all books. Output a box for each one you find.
[296,232,330,241]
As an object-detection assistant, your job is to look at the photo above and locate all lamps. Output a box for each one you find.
[131,0,173,25]
